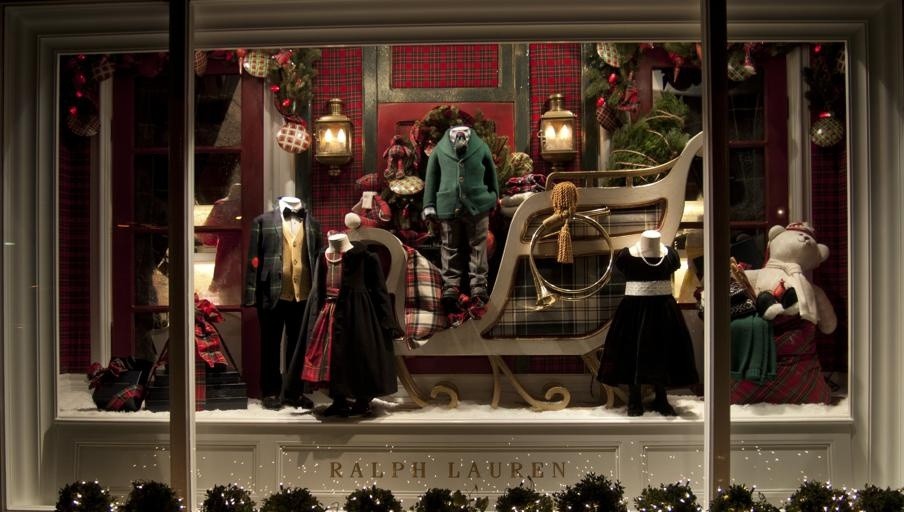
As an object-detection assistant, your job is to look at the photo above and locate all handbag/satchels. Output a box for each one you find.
[596,44,752,132]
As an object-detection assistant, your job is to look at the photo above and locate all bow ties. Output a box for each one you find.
[283,207,306,218]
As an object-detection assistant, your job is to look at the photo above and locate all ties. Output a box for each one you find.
[455,132,467,159]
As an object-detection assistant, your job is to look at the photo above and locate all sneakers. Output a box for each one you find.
[443,286,459,298]
[471,285,488,299]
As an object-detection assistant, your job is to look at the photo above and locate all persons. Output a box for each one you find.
[243,197,328,411]
[597,231,699,417]
[199,182,242,305]
[422,126,499,298]
[281,235,404,420]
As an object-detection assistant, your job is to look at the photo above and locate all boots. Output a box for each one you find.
[628,392,673,416]
[323,400,369,416]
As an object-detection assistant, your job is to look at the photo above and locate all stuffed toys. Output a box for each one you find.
[730,221,839,404]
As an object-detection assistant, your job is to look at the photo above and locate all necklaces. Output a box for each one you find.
[324,247,343,264]
[634,239,667,267]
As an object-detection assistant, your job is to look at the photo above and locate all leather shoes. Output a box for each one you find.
[263,395,314,408]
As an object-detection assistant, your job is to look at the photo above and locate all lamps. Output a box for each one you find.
[536,93,578,171]
[311,97,354,179]
